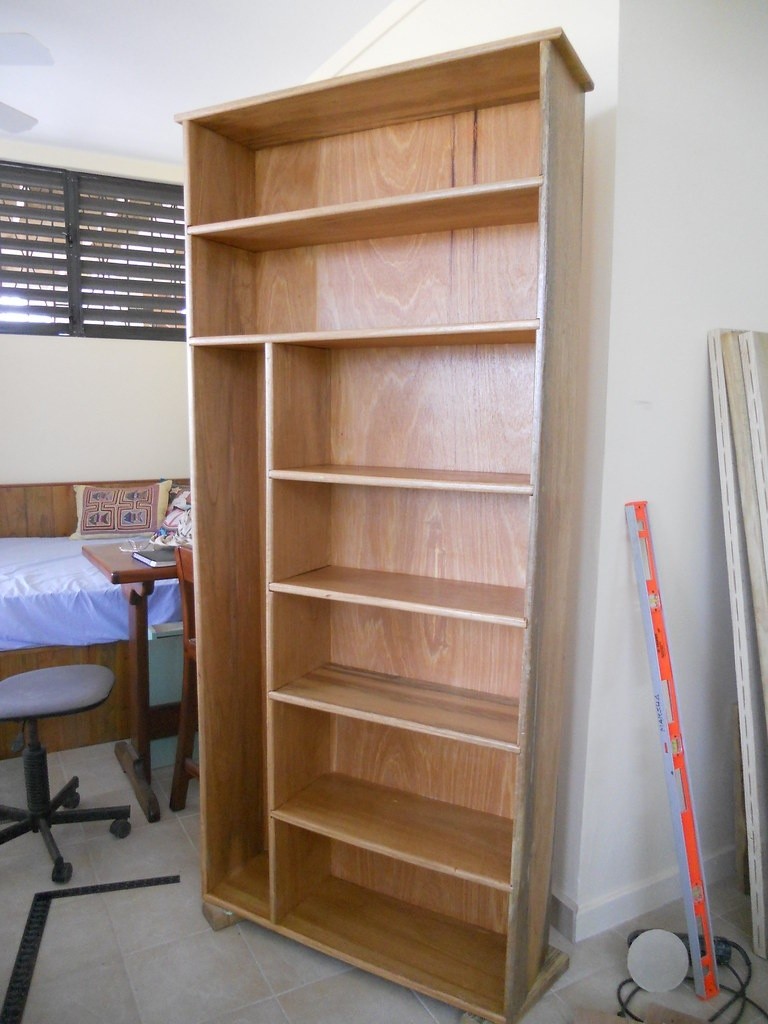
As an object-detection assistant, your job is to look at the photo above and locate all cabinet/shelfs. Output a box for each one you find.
[173,29,597,1023]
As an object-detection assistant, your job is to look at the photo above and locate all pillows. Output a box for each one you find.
[146,479,193,550]
[69,479,173,541]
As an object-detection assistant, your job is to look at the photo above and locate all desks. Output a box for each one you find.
[83,541,178,823]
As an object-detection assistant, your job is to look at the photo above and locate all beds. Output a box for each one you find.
[0,535,198,762]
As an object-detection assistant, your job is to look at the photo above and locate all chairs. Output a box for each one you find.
[169,546,199,812]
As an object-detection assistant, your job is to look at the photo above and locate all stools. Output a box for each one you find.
[0,665,131,885]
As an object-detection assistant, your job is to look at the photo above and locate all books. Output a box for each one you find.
[132,550,176,568]
